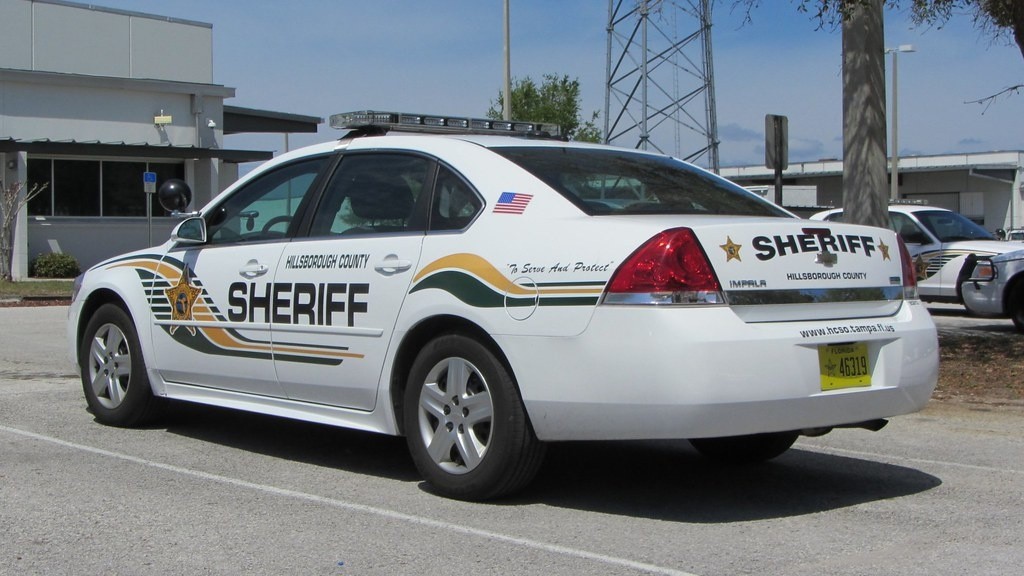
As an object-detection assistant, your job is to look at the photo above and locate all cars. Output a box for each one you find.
[809,199,1024,305]
[67,110,939,501]
[956,248,1024,332]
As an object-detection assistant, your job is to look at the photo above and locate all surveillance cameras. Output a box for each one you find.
[208,121,217,130]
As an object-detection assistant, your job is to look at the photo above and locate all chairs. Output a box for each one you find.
[341,173,415,234]
[900,224,915,240]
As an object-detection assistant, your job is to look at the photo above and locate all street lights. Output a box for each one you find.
[885,44,916,206]
